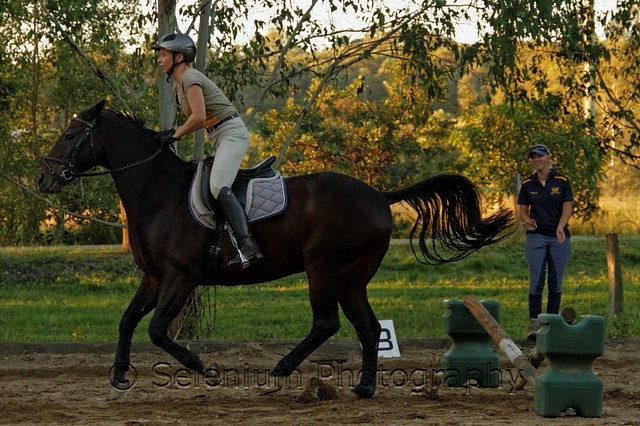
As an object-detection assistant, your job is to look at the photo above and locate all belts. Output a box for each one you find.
[205,112,238,135]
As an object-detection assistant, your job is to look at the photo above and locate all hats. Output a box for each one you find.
[529,145,550,156]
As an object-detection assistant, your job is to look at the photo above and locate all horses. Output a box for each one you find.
[35,99,521,402]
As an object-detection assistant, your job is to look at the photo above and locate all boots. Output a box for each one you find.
[217,187,263,272]
[527,318,538,340]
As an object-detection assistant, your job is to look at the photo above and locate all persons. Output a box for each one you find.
[153,35,265,269]
[517,143,573,339]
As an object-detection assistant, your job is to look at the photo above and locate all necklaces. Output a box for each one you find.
[538,176,547,183]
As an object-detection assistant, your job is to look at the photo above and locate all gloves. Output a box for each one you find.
[160,134,180,153]
[167,128,175,136]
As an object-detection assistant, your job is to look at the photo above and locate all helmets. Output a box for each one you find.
[151,33,196,63]
